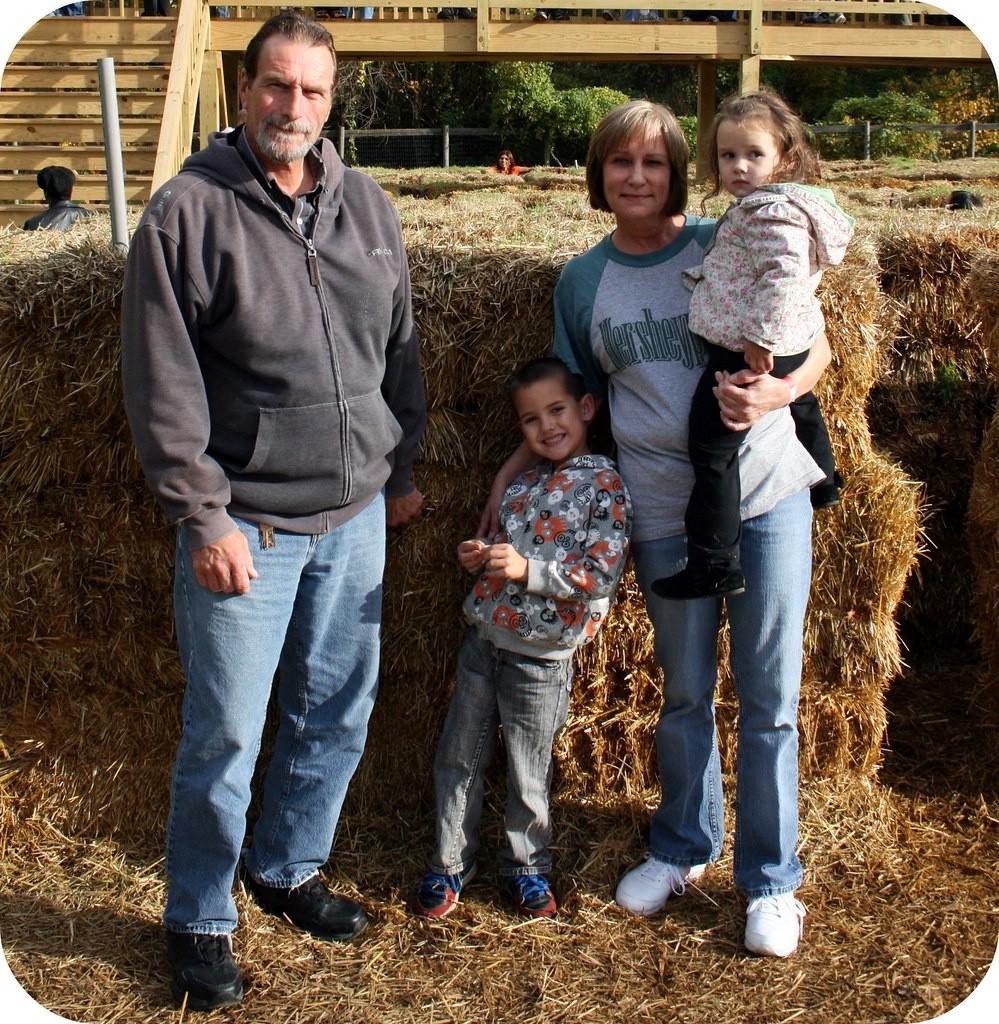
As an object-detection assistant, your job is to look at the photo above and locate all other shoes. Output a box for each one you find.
[48,4,847,25]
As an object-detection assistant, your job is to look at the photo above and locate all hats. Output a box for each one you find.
[37,165,78,194]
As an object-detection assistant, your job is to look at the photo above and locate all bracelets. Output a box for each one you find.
[782,375,798,405]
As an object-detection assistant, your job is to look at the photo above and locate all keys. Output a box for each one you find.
[260,524,276,550]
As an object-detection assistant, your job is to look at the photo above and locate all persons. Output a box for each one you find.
[121,11,423,1007]
[23,164,95,234]
[480,153,531,181]
[651,87,841,599]
[47,1,915,25]
[472,96,827,959]
[415,353,635,926]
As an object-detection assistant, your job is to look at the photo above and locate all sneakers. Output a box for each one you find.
[164,914,245,1011]
[416,859,478,918]
[812,475,845,506]
[616,846,706,917]
[744,890,806,956]
[507,873,558,919]
[651,560,746,599]
[246,873,369,942]
